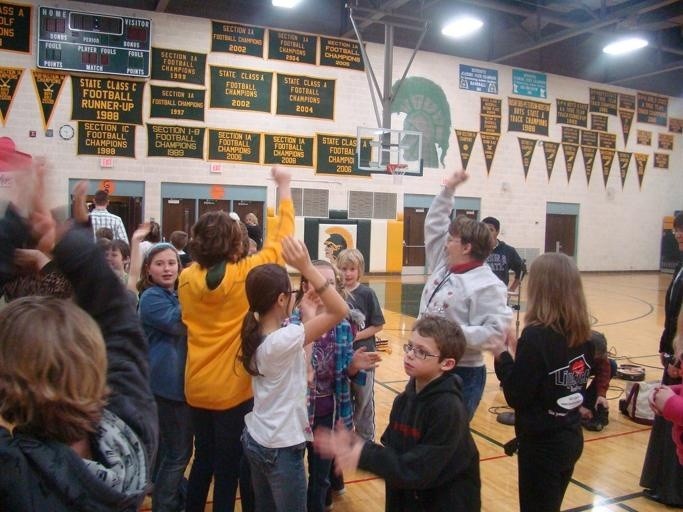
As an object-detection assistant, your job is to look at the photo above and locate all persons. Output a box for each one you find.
[336,247,385,443]
[178,165,295,512]
[416,169,515,421]
[315,314,481,512]
[285,261,382,512]
[235,213,264,262]
[579,328,617,421]
[89,190,197,300]
[323,227,353,271]
[481,217,526,304]
[482,253,595,512]
[0,200,77,300]
[638,210,683,507]
[240,239,348,512]
[138,244,192,512]
[0,227,158,511]
[648,303,683,470]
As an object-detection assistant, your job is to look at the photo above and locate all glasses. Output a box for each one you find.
[445,236,465,244]
[402,343,439,360]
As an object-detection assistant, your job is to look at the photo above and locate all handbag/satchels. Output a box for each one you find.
[625,378,661,425]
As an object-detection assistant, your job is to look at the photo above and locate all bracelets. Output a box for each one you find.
[316,280,329,294]
